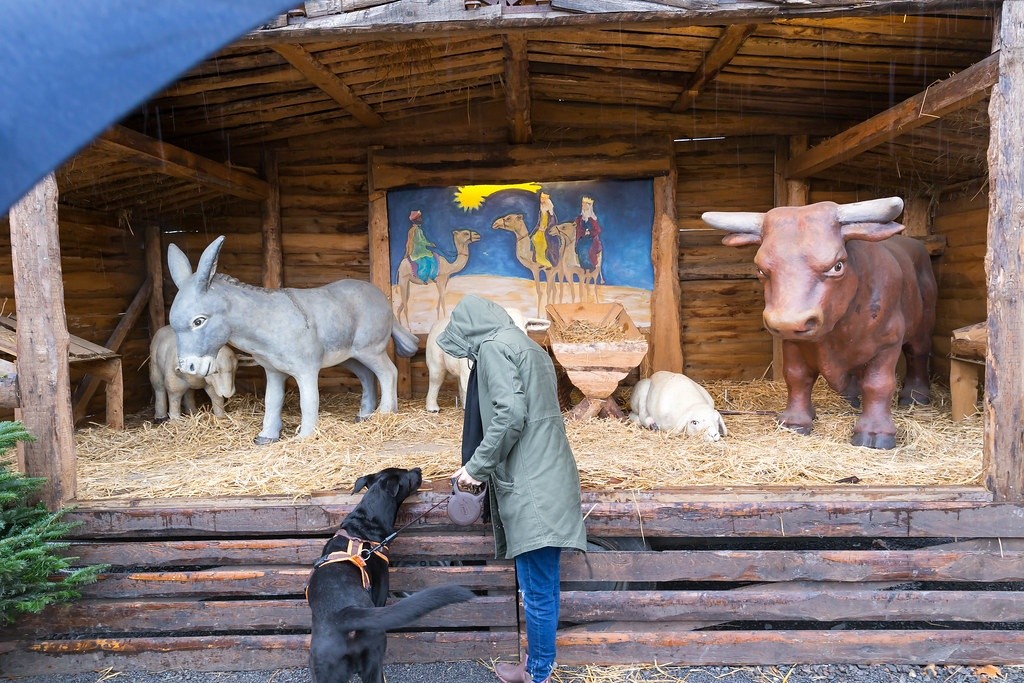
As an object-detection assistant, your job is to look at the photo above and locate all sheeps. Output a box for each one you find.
[629,370,728,442]
[425,312,551,414]
[147,324,261,424]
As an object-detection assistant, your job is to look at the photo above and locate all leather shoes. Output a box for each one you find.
[493,651,558,683]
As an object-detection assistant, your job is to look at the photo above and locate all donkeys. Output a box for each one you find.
[169,234,421,446]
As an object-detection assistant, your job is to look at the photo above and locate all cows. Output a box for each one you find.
[701,196,938,450]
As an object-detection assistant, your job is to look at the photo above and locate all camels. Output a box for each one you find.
[548,221,605,304]
[492,213,566,318]
[394,230,481,331]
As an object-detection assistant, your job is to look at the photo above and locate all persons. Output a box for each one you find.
[436,297,587,683]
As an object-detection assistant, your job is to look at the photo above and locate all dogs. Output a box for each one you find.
[304,467,477,683]
